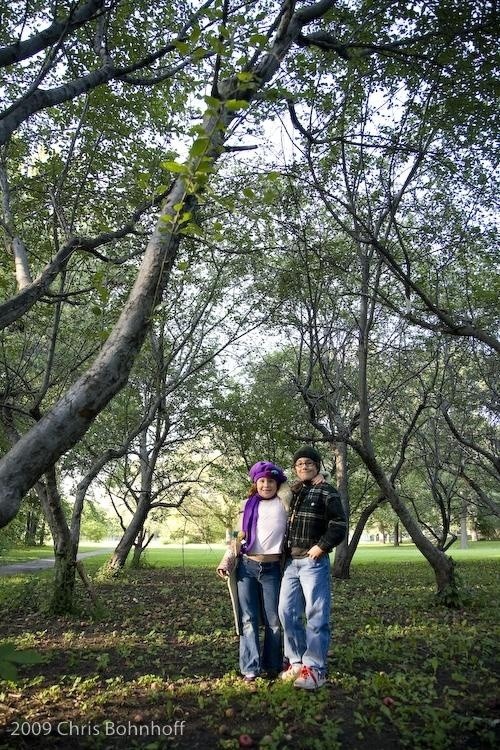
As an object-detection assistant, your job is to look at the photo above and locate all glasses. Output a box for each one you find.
[293,460,318,467]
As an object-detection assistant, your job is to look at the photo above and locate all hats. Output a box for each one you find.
[249,461,287,484]
[292,445,321,464]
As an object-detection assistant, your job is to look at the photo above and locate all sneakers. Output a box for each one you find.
[294,665,327,692]
[244,674,259,683]
[281,663,302,681]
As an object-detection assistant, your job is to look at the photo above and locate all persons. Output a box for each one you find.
[215,460,333,682]
[278,445,349,690]
